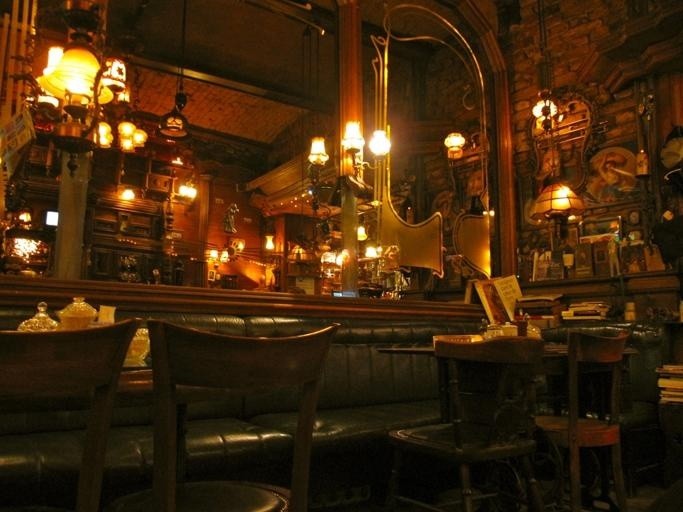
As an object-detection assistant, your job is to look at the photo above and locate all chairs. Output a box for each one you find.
[386,336,546,512]
[109,317,342,512]
[0,317,142,512]
[534,328,633,512]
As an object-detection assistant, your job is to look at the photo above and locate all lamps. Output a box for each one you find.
[265,235,275,250]
[444,119,466,161]
[210,248,229,269]
[323,227,378,269]
[156,0,194,140]
[342,120,392,199]
[531,0,559,134]
[307,137,341,210]
[11,0,148,154]
[528,182,585,253]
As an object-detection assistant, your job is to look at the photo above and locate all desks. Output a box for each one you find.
[376,342,638,427]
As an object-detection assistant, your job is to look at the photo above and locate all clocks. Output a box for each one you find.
[627,211,641,225]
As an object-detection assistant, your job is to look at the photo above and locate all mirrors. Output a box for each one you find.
[1,0,517,322]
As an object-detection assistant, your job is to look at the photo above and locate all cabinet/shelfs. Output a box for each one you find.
[0,111,207,289]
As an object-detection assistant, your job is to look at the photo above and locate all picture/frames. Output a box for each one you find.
[532,215,623,280]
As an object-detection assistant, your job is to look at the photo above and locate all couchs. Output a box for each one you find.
[0,273,672,511]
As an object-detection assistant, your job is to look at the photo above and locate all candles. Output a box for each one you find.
[637,150,648,175]
